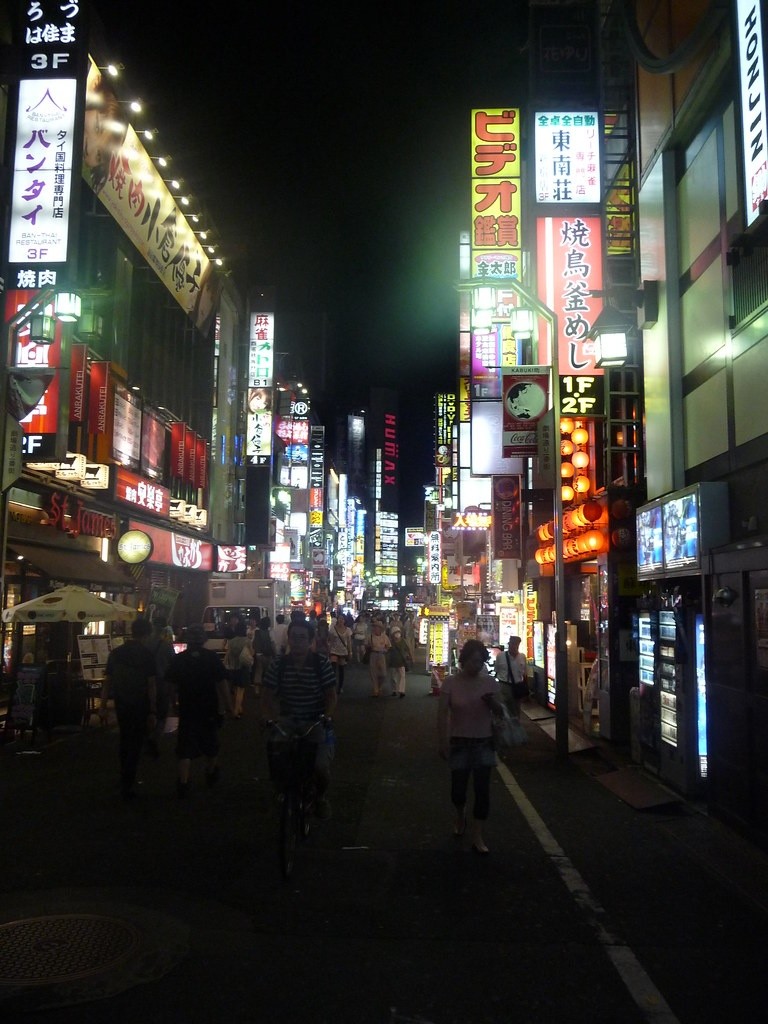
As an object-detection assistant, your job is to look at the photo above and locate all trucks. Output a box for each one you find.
[200,579,291,662]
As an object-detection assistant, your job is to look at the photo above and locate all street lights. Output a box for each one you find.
[1,285,111,446]
[423,484,451,494]
[453,274,569,770]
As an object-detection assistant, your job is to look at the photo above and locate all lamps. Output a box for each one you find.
[108,63,223,266]
[587,280,658,370]
[54,278,84,322]
[296,382,308,396]
[30,315,55,344]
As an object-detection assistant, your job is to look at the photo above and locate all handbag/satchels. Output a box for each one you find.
[402,654,410,665]
[512,681,530,699]
[362,646,371,664]
[493,711,512,747]
[345,653,351,661]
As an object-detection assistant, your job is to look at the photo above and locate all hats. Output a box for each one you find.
[371,621,383,627]
[391,627,400,635]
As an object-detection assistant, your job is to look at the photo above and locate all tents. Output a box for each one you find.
[2,586,136,722]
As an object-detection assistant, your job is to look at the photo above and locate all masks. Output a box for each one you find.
[394,633,400,638]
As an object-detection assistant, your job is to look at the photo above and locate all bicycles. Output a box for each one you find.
[256,714,333,881]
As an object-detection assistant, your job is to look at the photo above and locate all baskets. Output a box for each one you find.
[268,742,319,783]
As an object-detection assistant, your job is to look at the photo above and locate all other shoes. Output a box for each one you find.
[315,793,332,819]
[120,789,135,800]
[471,839,490,856]
[372,691,379,697]
[400,692,405,697]
[454,816,468,836]
[392,692,397,696]
[337,686,344,694]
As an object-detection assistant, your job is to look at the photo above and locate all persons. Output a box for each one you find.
[437,640,507,852]
[258,621,336,822]
[171,631,234,802]
[495,636,530,744]
[99,618,173,801]
[221,597,417,718]
